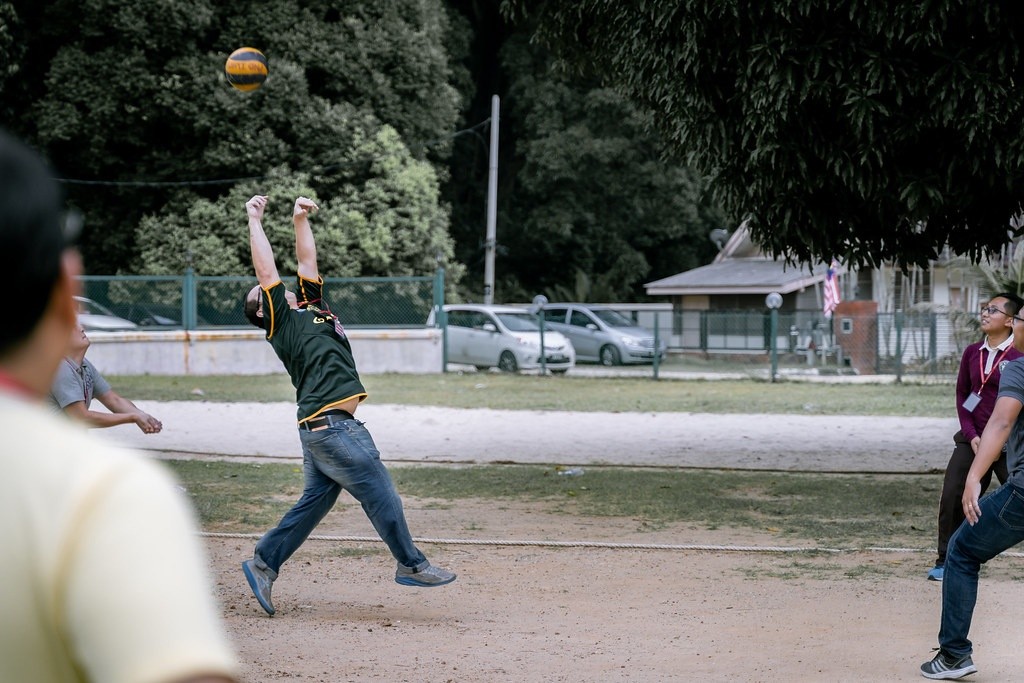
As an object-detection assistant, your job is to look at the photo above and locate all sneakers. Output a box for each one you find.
[921,654,977,679]
[395,566,455,586]
[242,561,276,615]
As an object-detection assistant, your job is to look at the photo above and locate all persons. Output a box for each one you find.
[243,194,457,615]
[50,320,163,435]
[928,294,1024,581]
[1,133,241,683]
[919,305,1023,681]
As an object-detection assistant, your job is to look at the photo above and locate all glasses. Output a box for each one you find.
[981,306,1012,317]
[1013,315,1024,325]
[61,214,85,253]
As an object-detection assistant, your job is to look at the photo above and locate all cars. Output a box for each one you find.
[107,303,208,328]
[525,303,665,366]
[425,304,577,376]
[72,295,137,333]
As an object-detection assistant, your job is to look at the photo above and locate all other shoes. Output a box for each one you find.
[928,567,944,581]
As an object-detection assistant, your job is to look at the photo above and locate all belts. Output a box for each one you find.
[300,414,351,430]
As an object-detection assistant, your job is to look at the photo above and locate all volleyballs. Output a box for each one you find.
[224,46,270,92]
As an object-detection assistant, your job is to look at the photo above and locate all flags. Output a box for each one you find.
[823,261,841,321]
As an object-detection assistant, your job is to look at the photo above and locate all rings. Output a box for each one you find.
[969,504,972,507]
